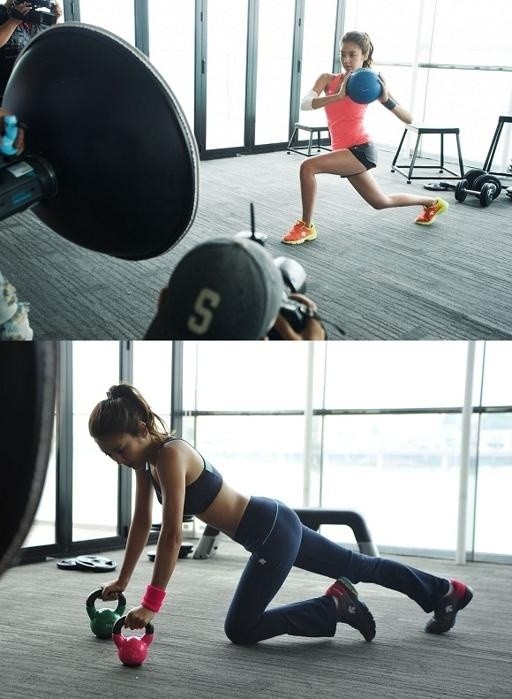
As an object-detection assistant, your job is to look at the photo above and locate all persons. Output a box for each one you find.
[1,108,26,158]
[88,384,476,647]
[284,29,450,246]
[263,291,327,340]
[1,0,63,100]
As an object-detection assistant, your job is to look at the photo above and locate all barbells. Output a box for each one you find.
[455,168,502,206]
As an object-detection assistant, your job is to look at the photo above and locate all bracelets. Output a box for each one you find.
[381,95,398,110]
[141,584,167,612]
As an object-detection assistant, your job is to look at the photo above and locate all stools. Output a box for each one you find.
[390,123,464,184]
[286,122,333,157]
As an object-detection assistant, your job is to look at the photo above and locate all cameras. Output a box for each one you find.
[234,231,306,340]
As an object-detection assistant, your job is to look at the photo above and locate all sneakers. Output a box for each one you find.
[280,218,318,245]
[324,576,376,643]
[422,580,475,633]
[414,197,450,225]
